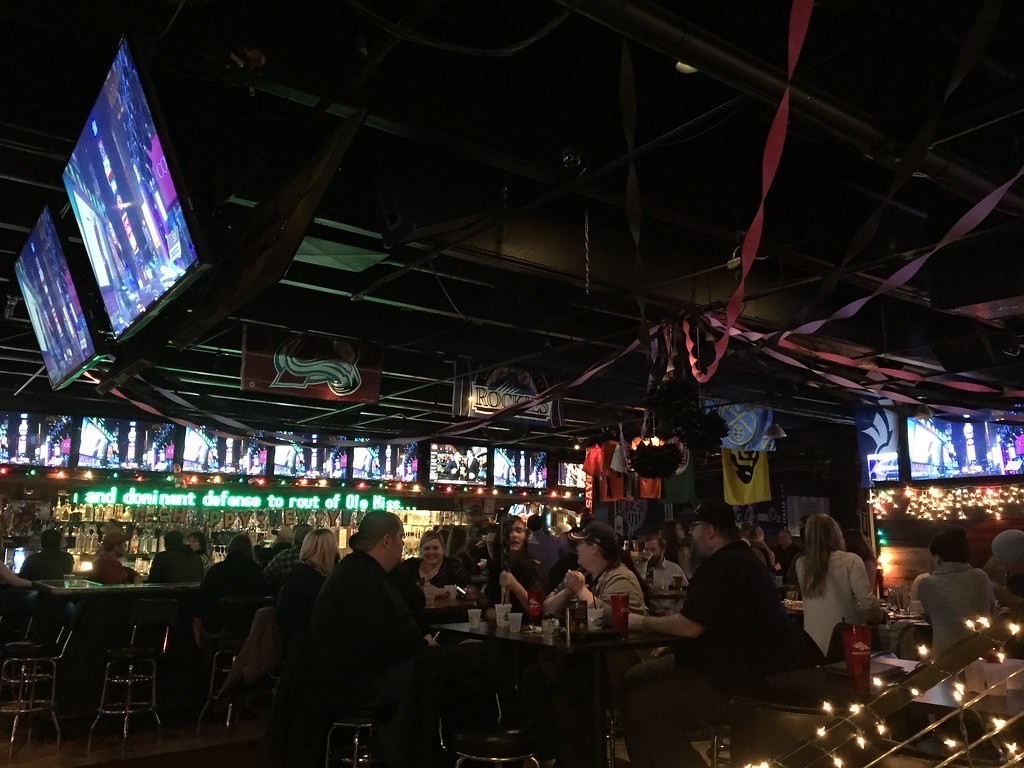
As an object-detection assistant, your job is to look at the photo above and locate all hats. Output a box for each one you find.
[568,521,618,546]
[677,500,736,528]
[102,532,131,546]
[992,529,1024,559]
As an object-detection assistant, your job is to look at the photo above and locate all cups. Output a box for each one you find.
[671,575,682,589]
[840,626,873,692]
[494,603,512,626]
[135,558,143,572]
[467,609,482,628]
[775,575,782,586]
[636,560,648,580]
[444,585,458,600]
[887,583,924,617]
[424,586,436,605]
[506,613,523,632]
[542,620,554,638]
[63,575,75,582]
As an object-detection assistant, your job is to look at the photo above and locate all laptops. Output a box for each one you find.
[5,548,39,574]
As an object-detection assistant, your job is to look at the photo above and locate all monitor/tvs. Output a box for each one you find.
[0,407,585,492]
[12,202,108,392]
[57,30,221,343]
[867,414,1024,487]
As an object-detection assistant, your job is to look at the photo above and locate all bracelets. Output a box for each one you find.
[640,615,648,634]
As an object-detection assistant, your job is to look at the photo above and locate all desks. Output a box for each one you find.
[0,565,931,730]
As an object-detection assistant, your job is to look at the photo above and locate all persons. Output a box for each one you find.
[911,527,1024,646]
[0,510,885,768]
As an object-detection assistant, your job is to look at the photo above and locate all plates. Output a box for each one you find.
[784,601,804,611]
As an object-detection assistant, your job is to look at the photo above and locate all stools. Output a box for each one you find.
[0,599,552,768]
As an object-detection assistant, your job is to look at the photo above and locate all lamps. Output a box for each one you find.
[674,324,733,455]
[627,412,683,479]
[763,423,788,438]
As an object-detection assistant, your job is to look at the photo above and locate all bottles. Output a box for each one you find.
[703,724,732,768]
[48,494,466,561]
[604,707,626,768]
[960,657,1024,694]
